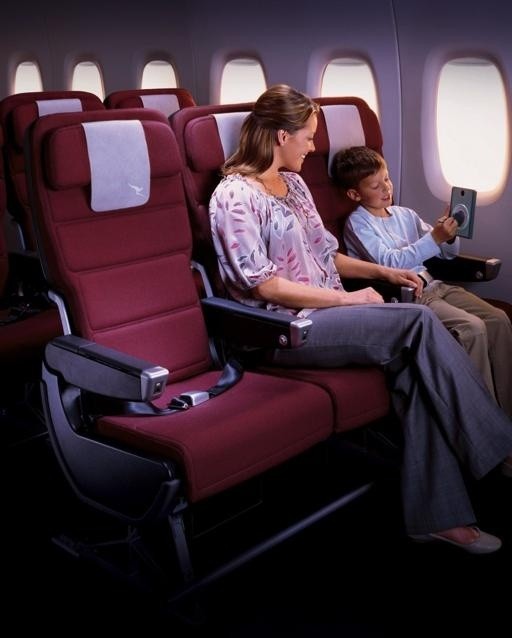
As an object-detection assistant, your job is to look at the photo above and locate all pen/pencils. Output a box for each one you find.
[438,220,444,223]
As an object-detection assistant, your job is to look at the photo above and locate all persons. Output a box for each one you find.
[330,146,512,416]
[209,84,512,555]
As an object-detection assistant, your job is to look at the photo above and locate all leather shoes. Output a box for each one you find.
[411,522,504,557]
